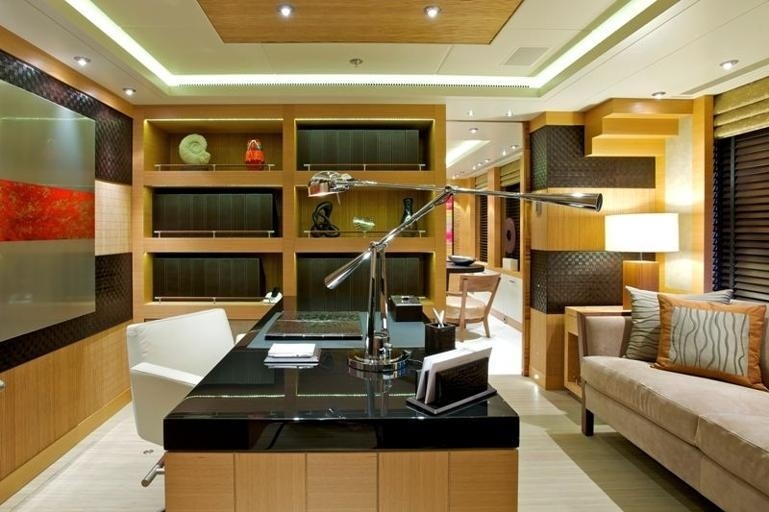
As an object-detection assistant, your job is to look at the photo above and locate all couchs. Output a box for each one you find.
[580,315,769,512]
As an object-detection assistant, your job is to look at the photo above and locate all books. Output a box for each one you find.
[267,342,317,358]
[263,347,322,365]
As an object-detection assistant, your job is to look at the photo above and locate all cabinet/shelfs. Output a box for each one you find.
[132,103,447,327]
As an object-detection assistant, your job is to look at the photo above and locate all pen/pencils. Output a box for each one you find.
[432,308,445,327]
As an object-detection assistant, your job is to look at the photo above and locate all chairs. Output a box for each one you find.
[446,274,502,344]
[126,308,247,488]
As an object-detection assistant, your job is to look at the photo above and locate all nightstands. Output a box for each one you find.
[563,305,632,400]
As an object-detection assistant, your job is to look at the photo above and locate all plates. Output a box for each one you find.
[448,255,477,265]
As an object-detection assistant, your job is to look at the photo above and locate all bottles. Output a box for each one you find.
[400,198,418,237]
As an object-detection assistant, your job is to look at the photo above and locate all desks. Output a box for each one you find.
[446,261,485,292]
[162,296,519,511]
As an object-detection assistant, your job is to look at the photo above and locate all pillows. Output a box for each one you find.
[623,284,769,391]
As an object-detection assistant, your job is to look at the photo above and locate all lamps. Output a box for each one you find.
[307,171,602,380]
[605,212,679,308]
